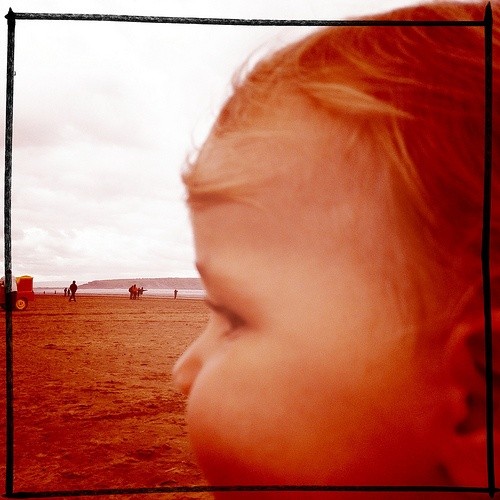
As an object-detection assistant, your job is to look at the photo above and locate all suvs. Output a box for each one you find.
[0,275,34,312]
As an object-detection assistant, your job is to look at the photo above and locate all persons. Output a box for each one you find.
[68,281,77,301]
[64,288,70,296]
[0,270,17,311]
[174,289,178,299]
[172,0,500,500]
[129,284,147,300]
